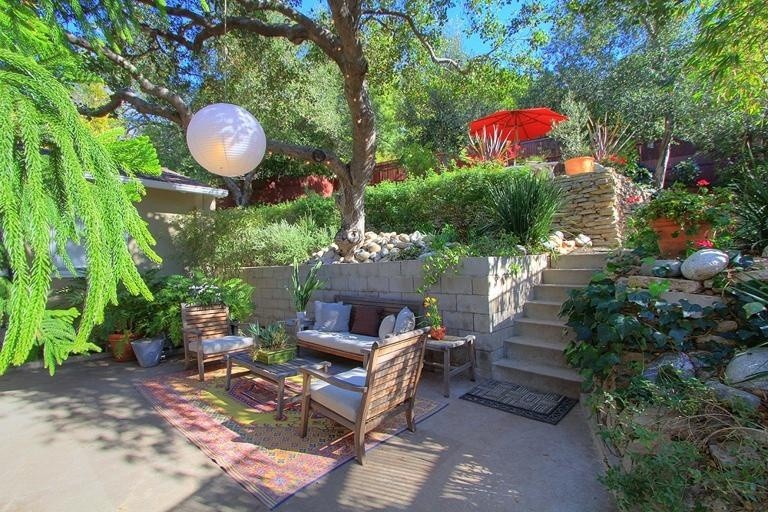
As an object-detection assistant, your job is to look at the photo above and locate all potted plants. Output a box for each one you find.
[50,268,177,369]
[546,94,604,177]
[282,252,330,333]
[248,321,296,366]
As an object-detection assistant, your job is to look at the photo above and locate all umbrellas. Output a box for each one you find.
[467,108,567,154]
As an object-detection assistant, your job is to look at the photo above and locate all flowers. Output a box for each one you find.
[622,177,738,262]
[414,296,448,331]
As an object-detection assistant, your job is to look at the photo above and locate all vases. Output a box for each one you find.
[431,324,448,342]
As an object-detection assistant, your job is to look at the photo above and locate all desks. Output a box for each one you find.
[222,348,334,422]
[420,331,477,398]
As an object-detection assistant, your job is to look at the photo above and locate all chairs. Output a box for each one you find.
[298,326,433,465]
[180,300,258,382]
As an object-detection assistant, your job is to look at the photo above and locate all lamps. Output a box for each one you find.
[185,1,267,180]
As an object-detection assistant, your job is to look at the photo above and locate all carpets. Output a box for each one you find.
[127,352,451,511]
[459,375,581,427]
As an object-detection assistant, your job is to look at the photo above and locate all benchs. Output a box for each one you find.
[296,294,424,371]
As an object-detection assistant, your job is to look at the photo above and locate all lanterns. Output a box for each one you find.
[185,102,267,177]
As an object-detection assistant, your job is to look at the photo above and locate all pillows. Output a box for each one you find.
[312,298,420,338]
